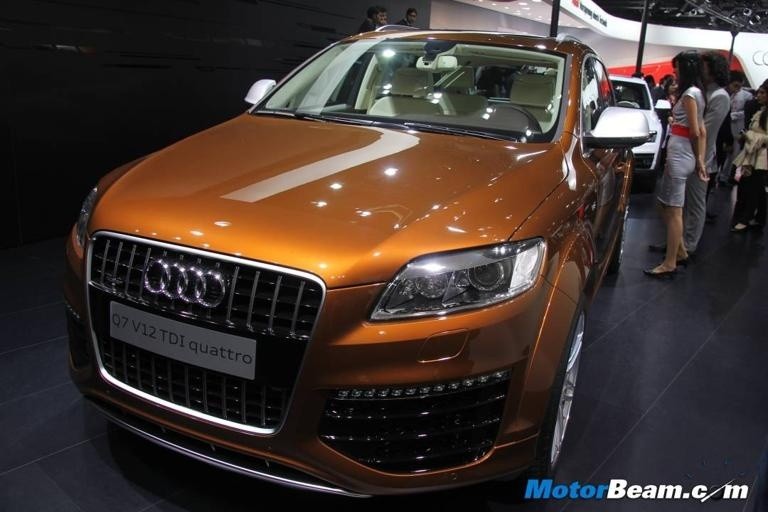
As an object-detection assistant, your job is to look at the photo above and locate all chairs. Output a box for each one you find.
[620,91,637,108]
[366,67,557,133]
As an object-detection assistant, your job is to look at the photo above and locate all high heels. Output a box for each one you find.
[643,268,678,280]
[677,255,689,269]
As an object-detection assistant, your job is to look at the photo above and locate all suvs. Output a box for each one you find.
[57,23,657,512]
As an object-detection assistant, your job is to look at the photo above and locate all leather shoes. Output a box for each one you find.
[729,222,747,232]
[649,240,666,253]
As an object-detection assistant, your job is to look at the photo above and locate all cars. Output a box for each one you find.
[604,74,679,193]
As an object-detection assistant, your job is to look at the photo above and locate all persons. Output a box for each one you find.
[357,7,385,35]
[396,6,418,27]
[647,51,732,267]
[376,7,388,31]
[627,67,768,234]
[636,49,712,279]
